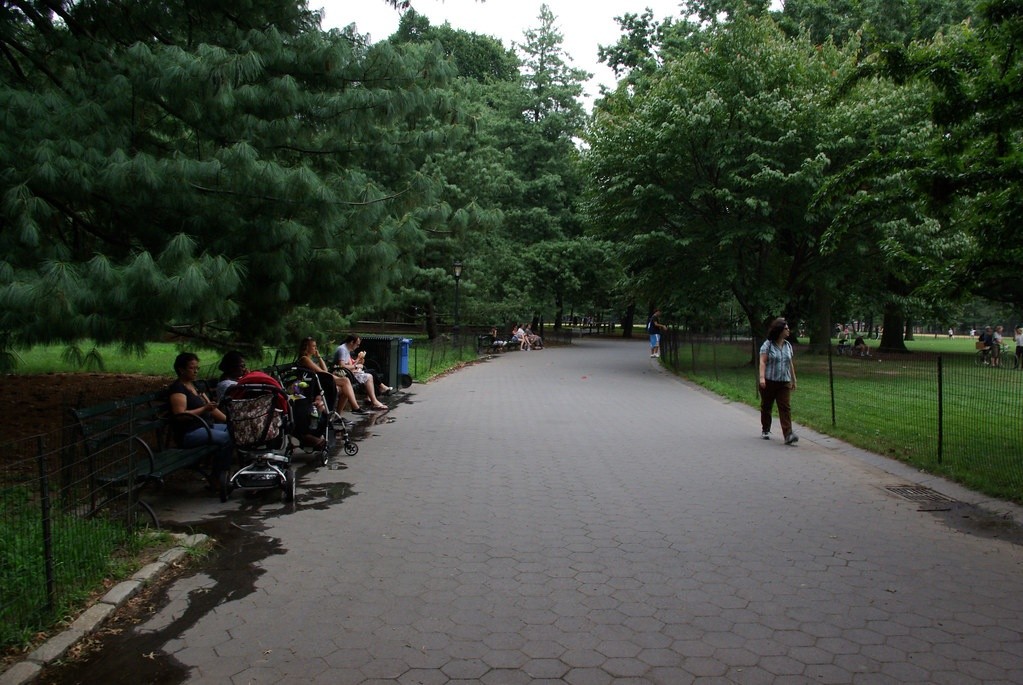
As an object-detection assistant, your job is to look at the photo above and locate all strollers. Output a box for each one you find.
[218,371,296,505]
[282,367,358,468]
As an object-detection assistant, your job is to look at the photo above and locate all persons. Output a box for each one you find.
[581,315,599,327]
[969,328,976,340]
[165,352,239,497]
[854,335,872,357]
[510,323,525,351]
[646,308,668,359]
[517,323,533,351]
[990,325,1007,368]
[758,317,799,444]
[332,334,389,410]
[835,322,841,339]
[979,325,994,365]
[844,326,849,340]
[295,336,370,425]
[525,323,545,350]
[214,352,328,453]
[488,326,509,351]
[1011,324,1023,370]
[340,339,394,404]
[948,327,954,340]
[875,325,883,340]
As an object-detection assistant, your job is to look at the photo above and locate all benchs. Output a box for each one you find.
[476,332,543,354]
[67,355,383,533]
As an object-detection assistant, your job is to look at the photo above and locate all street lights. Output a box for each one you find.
[452,260,463,328]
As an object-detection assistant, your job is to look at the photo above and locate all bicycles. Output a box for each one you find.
[974,343,1020,369]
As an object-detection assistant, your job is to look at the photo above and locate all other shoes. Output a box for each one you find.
[860,353,873,356]
[364,396,388,410]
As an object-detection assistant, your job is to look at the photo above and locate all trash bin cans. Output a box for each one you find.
[355,336,415,396]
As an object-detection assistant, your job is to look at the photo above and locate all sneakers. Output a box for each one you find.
[761,431,772,439]
[651,353,659,358]
[380,387,393,393]
[785,433,799,444]
[351,408,369,415]
[332,417,352,425]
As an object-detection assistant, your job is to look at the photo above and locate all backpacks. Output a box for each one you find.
[647,317,655,332]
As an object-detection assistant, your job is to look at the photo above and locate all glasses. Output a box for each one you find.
[784,327,789,330]
[355,343,360,346]
[184,366,200,370]
